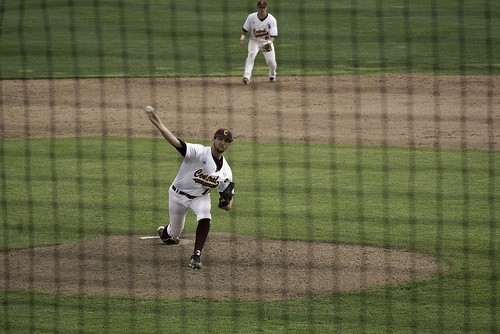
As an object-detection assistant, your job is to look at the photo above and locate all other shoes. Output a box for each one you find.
[268,76,276,82]
[242,77,250,85]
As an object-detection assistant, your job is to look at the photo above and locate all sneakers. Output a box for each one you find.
[157,226,179,245]
[189,255,202,269]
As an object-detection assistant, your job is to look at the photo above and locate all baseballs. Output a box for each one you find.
[144,105,154,113]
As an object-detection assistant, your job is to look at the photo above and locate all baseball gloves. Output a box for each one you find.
[217,182,236,208]
[263,41,273,53]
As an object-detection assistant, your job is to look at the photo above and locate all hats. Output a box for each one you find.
[214,128,234,143]
[257,0,267,8]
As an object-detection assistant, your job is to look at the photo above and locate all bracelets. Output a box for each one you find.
[240,34,245,41]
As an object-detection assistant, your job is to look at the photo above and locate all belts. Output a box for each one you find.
[172,185,197,199]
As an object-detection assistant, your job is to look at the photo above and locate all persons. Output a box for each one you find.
[240,0,278,84]
[147,111,235,269]
